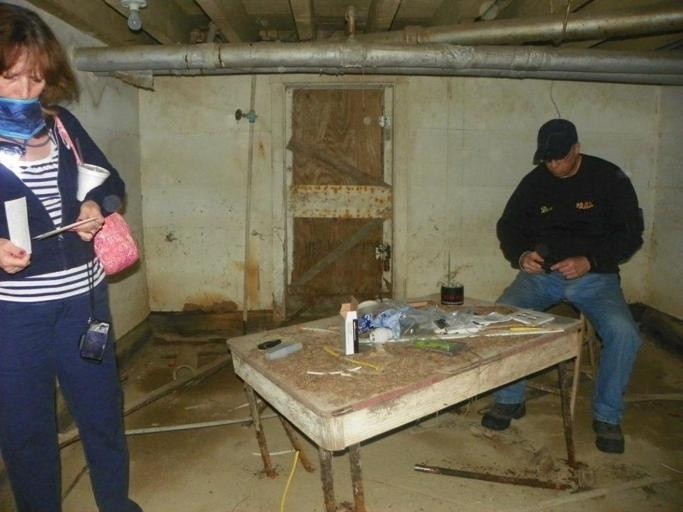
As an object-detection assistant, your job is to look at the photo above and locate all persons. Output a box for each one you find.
[481,119,645,453]
[0,3,142,511]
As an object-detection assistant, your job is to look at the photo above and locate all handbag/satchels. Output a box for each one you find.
[93,211,139,275]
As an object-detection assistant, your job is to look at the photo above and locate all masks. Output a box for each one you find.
[1,101,48,140]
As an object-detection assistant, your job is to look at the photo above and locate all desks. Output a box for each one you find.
[226,293,582,512]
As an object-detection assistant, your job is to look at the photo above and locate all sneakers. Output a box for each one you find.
[592,418,624,454]
[481,401,526,430]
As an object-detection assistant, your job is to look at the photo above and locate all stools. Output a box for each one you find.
[478,304,603,422]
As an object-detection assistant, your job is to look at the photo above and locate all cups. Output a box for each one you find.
[76,163,109,202]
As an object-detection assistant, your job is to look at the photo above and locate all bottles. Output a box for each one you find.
[345,311,358,354]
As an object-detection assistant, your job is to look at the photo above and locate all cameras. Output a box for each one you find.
[77,315,110,365]
[537,258,556,275]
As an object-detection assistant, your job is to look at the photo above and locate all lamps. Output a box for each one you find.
[121,0,147,31]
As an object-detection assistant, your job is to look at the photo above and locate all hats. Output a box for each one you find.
[533,119,577,165]
